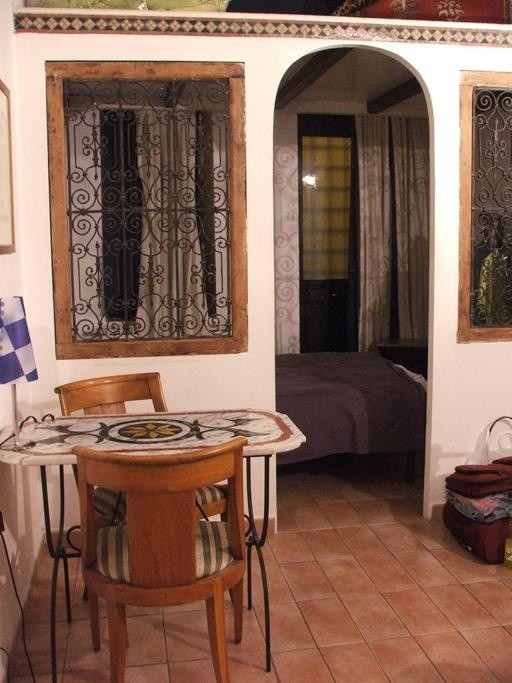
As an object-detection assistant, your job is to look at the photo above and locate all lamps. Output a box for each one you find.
[1,296,39,452]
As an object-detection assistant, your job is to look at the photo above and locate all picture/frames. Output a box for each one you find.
[0,80,15,254]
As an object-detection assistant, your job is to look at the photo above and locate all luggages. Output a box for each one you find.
[443,456,511,566]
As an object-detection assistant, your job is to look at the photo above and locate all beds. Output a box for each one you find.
[277,351,427,483]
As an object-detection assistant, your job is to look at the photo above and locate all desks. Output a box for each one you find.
[1,410,306,683]
